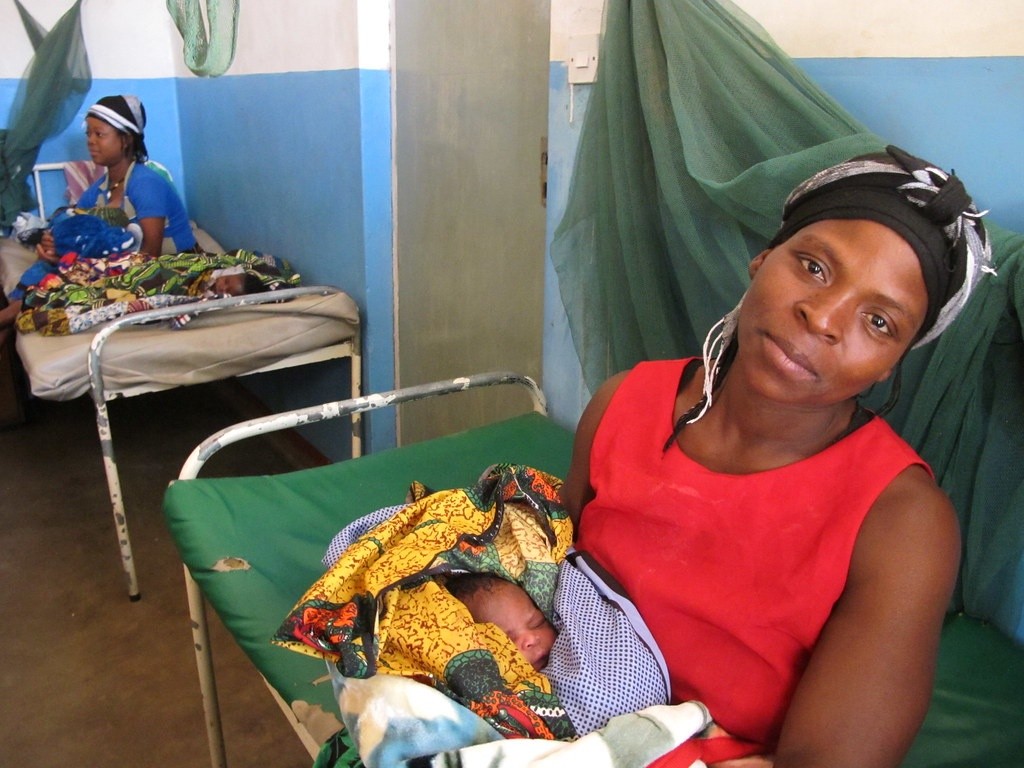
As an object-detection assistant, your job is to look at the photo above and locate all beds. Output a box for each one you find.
[164,371,1023,768]
[0,162,364,603]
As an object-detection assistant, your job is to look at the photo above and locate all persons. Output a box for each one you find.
[0,95,201,346]
[210,274,261,295]
[446,573,555,674]
[314,144,990,768]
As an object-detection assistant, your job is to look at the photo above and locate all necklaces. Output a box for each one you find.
[107,178,123,200]
